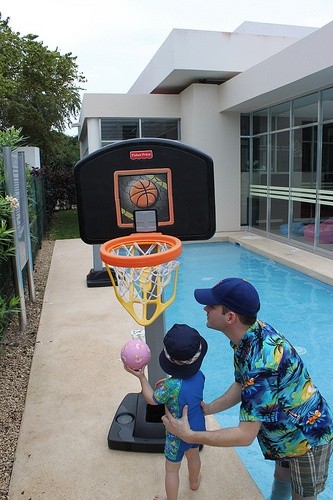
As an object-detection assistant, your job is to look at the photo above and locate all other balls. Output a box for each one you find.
[120,339,151,370]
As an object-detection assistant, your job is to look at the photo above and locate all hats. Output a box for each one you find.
[194,278,261,317]
[159,323,208,378]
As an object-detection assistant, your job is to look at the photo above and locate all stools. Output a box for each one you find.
[302,216,333,244]
[280,223,304,236]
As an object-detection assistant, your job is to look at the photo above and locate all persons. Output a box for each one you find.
[124,324,208,500]
[161,277,333,500]
